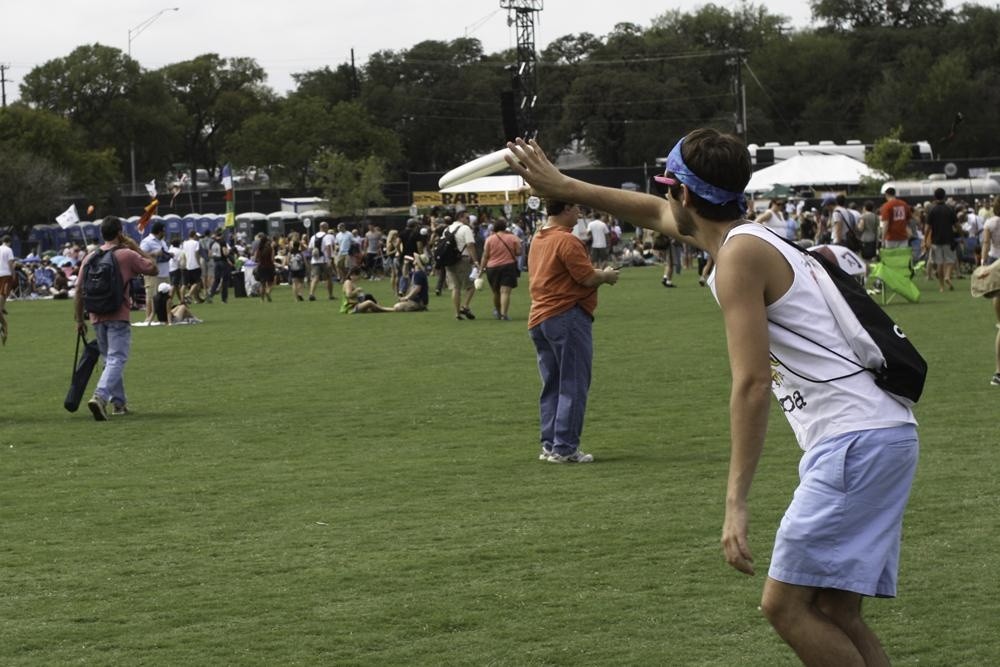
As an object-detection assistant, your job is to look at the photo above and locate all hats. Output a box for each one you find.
[414,253,429,272]
[312,247,319,260]
[820,197,836,207]
[158,282,170,294]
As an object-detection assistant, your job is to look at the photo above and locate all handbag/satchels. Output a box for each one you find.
[840,229,857,249]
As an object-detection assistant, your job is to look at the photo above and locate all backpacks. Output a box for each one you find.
[81,245,129,314]
[717,223,928,408]
[288,253,303,270]
[311,233,326,257]
[436,225,469,266]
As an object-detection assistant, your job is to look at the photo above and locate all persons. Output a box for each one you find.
[528,198,622,464]
[990,331,1000,386]
[73,215,159,421]
[503,129,928,667]
[0,187,1000,329]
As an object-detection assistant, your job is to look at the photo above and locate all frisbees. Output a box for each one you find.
[438,144,536,190]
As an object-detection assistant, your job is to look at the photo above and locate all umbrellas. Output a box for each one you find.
[744,155,891,193]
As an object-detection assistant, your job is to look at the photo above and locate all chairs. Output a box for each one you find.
[881,249,924,304]
[8,269,30,300]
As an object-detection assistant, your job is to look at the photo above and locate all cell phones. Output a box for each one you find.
[611,264,623,269]
[118,230,123,240]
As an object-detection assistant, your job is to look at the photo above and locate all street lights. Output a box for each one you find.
[127,7,179,196]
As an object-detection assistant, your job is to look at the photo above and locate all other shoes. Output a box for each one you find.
[990,373,1000,385]
[181,290,336,302]
[188,317,203,323]
[455,315,464,320]
[662,279,676,287]
[460,306,475,319]
[500,315,510,320]
[494,310,500,319]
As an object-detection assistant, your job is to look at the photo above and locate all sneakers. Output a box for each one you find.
[88,395,108,421]
[112,406,127,415]
[539,448,554,460]
[548,449,593,463]
